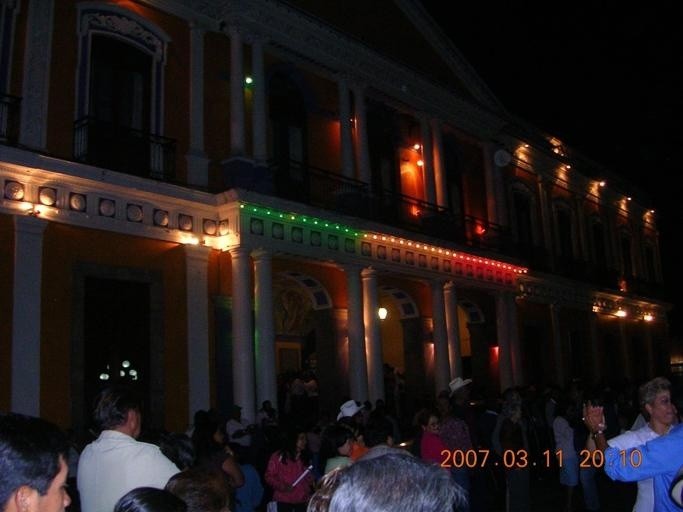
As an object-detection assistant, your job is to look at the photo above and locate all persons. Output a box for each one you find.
[0,374,683,512]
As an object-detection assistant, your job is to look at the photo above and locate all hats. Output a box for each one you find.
[449,376,473,398]
[336,400,364,421]
